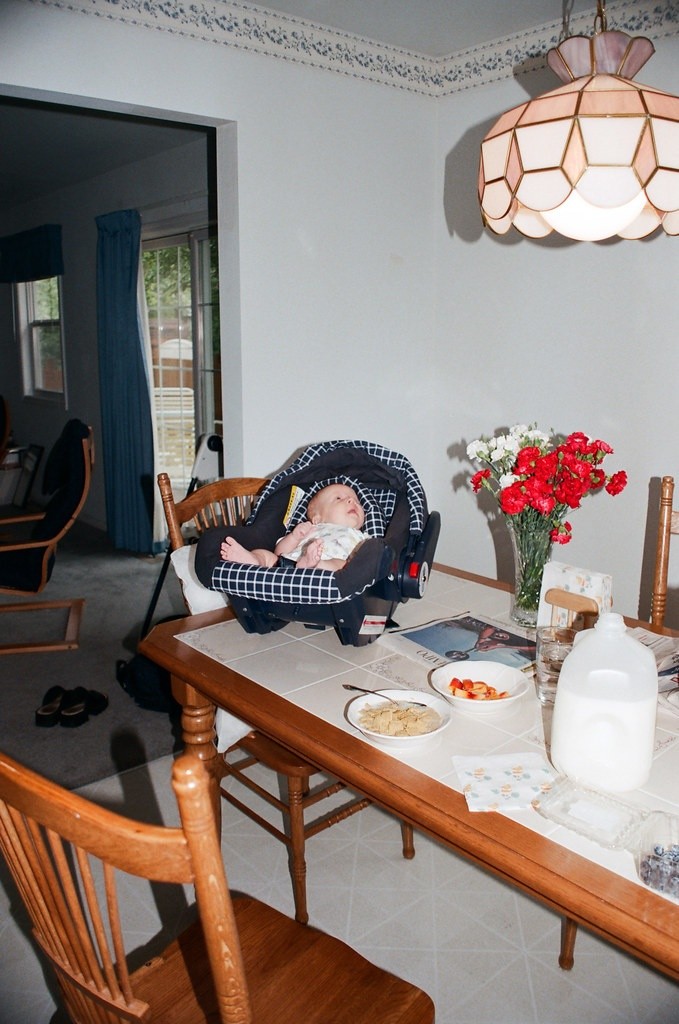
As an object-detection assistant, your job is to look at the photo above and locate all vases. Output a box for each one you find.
[505,521,562,629]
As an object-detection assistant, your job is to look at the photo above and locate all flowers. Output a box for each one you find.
[466,423,628,610]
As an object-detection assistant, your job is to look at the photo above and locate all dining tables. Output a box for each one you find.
[137,560,679,987]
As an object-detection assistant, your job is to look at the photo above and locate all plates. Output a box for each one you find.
[430,659,531,714]
[347,689,453,746]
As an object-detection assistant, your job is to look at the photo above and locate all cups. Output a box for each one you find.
[535,626,580,707]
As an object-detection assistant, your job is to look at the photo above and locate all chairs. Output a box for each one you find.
[158,471,415,926]
[650,476,679,632]
[0,751,438,1024]
[0,418,96,655]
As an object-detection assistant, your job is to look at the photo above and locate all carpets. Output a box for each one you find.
[0,516,218,792]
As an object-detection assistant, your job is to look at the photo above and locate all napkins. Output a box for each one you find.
[450,752,558,813]
[535,561,613,643]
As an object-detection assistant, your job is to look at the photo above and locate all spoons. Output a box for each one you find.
[343,685,427,709]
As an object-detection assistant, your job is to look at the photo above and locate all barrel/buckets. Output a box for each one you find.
[549,613,659,795]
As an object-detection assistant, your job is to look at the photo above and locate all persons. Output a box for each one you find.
[474,624,536,651]
[220,484,373,572]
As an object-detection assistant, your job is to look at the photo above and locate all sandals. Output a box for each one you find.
[59,687,109,728]
[35,685,74,727]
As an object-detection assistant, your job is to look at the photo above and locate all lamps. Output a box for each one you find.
[476,0,679,242]
